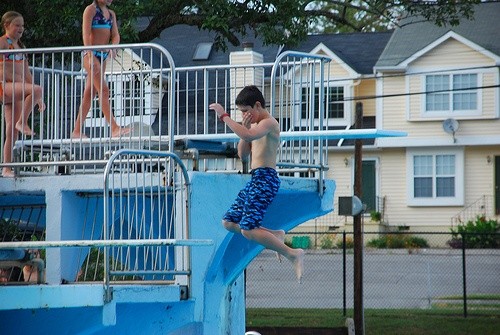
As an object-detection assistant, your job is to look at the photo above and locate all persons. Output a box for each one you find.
[0,12,45,178]
[71,0,132,140]
[209,86,305,284]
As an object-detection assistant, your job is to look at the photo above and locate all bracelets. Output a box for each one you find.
[220,114,229,120]
